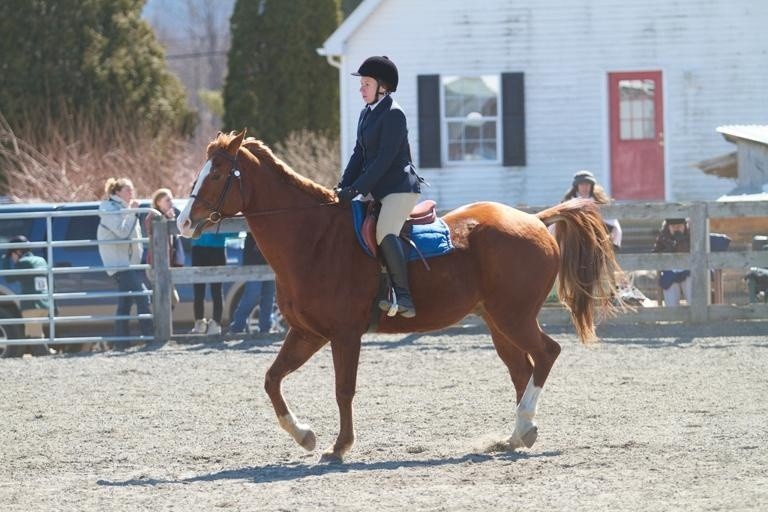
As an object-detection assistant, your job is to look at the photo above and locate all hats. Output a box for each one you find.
[666,219,686,225]
[572,171,595,187]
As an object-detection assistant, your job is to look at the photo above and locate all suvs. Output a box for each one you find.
[0,200,291,360]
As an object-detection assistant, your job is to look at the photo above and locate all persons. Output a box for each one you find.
[143,186,182,334]
[330,55,430,320]
[2,233,61,359]
[96,177,154,353]
[229,227,276,334]
[188,231,227,336]
[652,218,731,307]
[559,169,623,250]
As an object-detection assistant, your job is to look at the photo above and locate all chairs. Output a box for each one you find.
[651,233,731,304]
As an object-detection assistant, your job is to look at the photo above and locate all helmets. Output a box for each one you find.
[350,56,398,91]
[6,236,32,254]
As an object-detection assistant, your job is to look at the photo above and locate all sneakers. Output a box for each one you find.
[191,318,221,335]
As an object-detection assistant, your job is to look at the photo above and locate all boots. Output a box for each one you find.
[378,234,416,318]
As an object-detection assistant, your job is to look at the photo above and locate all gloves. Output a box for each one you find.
[333,182,358,207]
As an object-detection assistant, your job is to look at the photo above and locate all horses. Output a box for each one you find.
[174,124,638,463]
